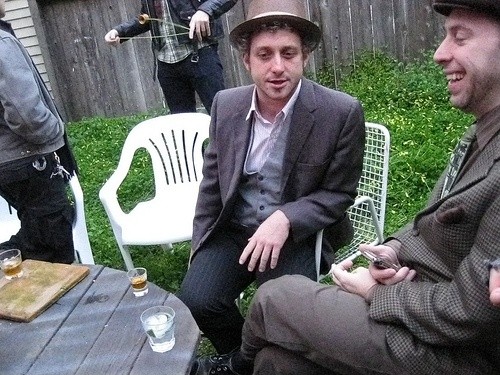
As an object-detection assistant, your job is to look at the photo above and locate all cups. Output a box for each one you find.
[0,248,23,279]
[127,267,148,296]
[140,306,176,353]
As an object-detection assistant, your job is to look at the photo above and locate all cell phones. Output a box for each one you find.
[362,249,400,271]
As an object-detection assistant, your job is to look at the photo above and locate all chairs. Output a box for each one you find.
[0,170,96,265]
[315,122,390,284]
[98,111,211,272]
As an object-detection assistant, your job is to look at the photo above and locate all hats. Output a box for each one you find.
[432,0,500,16]
[228,0,322,53]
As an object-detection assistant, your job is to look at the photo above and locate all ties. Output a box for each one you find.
[441,123,476,200]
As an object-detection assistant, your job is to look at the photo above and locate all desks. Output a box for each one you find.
[0,263,203,375]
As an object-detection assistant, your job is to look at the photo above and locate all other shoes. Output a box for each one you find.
[186,345,254,375]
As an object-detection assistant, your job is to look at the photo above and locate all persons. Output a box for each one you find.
[176,0,365,354]
[105,0,238,160]
[187,0,500,375]
[0,0,77,265]
